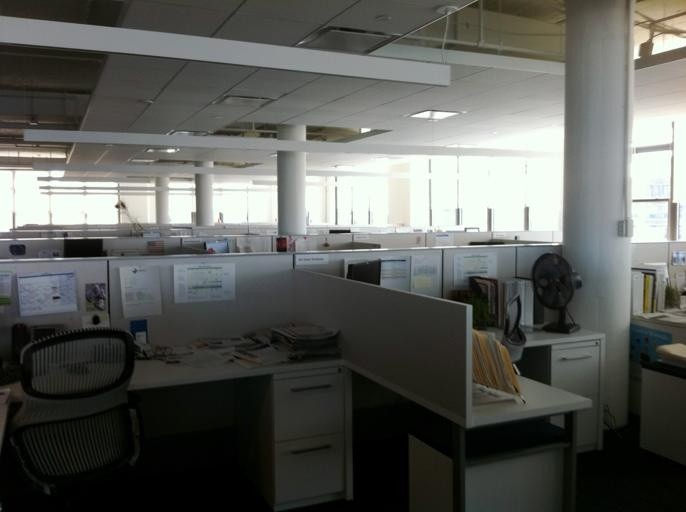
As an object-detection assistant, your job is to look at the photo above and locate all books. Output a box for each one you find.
[195,321,343,369]
[453,275,535,400]
[629,262,670,316]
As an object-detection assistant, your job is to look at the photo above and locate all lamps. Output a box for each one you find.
[639,22,656,57]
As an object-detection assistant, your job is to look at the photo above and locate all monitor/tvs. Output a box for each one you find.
[64,238,103,258]
[347,260,381,286]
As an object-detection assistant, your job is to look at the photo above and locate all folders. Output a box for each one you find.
[632,273,644,315]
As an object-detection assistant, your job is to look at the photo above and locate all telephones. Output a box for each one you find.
[11,323,64,364]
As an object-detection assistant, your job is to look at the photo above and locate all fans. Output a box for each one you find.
[532,252,585,334]
